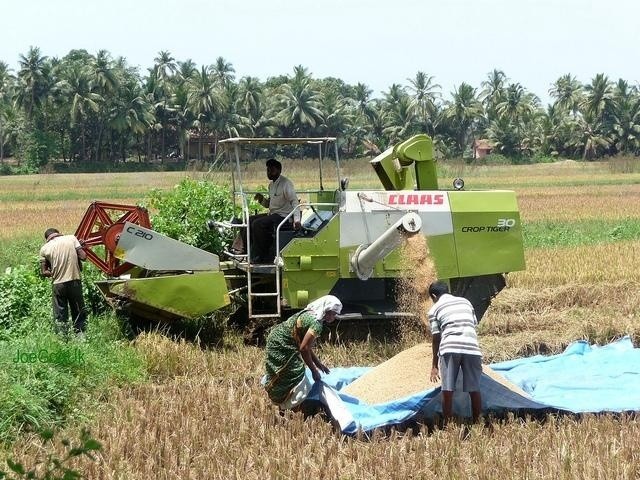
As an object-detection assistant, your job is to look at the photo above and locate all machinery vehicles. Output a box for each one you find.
[66,129,533,344]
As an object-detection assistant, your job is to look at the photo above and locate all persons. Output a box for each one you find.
[40,228,87,333]
[428,280,483,426]
[265,295,343,416]
[240,159,301,263]
